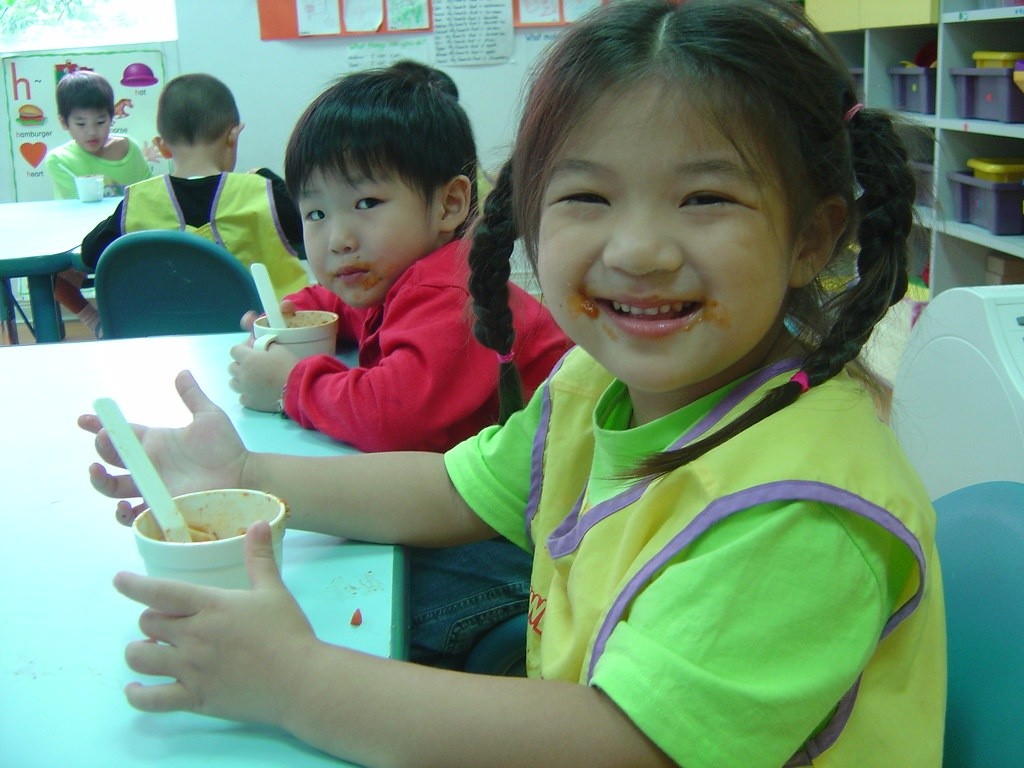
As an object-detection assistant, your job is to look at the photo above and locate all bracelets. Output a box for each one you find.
[276,380,293,423]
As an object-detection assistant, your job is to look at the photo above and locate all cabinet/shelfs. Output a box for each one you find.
[790,0,1024,306]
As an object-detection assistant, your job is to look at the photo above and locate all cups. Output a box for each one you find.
[131,485,287,600]
[74,174,104,203]
[253,310,339,371]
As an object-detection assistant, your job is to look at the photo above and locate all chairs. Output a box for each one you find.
[890,283,1024,768]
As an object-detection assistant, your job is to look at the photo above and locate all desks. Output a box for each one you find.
[0,331,408,768]
[0,196,124,347]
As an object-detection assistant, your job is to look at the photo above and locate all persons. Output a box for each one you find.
[81,72,314,315]
[226,57,575,679]
[76,0,950,768]
[43,71,154,339]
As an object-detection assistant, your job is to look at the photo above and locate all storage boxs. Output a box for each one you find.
[972,51,1024,68]
[887,67,937,114]
[907,160,934,208]
[848,68,864,103]
[967,157,1024,183]
[988,255,1024,285]
[948,171,1024,236]
[948,68,1024,123]
